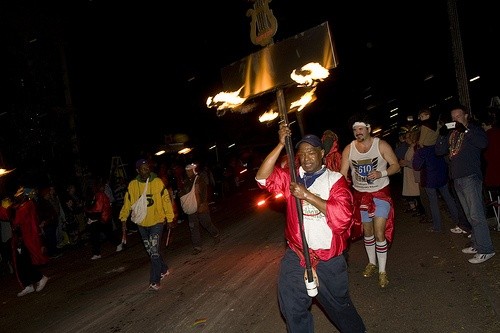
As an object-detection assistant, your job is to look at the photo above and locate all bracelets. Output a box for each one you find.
[380,170,388,177]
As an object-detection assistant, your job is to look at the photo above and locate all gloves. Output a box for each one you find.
[451,120,467,133]
[437,120,450,133]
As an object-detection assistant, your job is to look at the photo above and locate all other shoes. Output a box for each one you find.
[90,255,101,260]
[17,285,34,297]
[35,274,48,292]
[160,270,170,280]
[213,232,220,245]
[450,227,467,233]
[405,206,433,223]
[148,282,160,290]
[362,262,378,277]
[192,249,202,255]
[462,247,477,253]
[468,252,495,263]
[116,241,122,251]
[378,272,389,288]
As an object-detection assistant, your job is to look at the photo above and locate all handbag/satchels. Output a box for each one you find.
[131,177,149,224]
[180,174,198,215]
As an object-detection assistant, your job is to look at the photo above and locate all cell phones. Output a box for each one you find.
[445,122,456,129]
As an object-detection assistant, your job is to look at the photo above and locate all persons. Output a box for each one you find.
[321,105,500,287]
[255,123,367,333]
[0,159,220,296]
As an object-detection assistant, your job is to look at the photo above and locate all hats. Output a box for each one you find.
[295,134,323,148]
[136,159,152,168]
